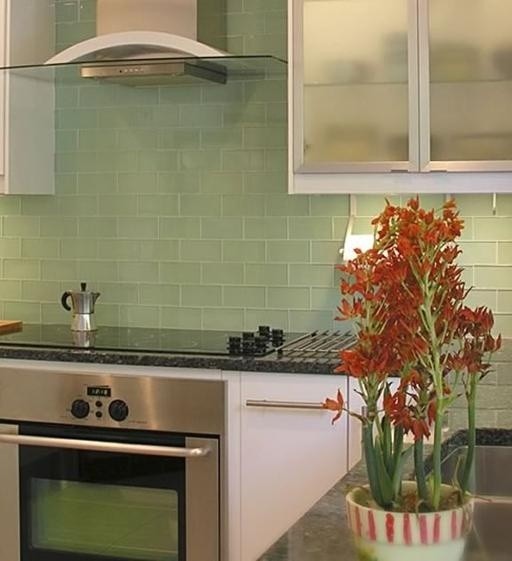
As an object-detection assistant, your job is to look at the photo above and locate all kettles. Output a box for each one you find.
[60,281,102,333]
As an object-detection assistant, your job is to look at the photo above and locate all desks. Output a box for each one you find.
[249,423,512,561]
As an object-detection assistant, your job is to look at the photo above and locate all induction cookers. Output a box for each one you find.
[1,323,298,359]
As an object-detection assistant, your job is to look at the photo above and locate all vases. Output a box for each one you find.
[346,478,475,561]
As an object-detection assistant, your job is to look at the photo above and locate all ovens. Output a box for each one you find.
[0,357,228,561]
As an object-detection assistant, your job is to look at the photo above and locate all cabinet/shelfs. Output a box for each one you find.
[237,375,421,560]
[286,1,512,198]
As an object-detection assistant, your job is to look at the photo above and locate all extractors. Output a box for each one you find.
[44,0,231,88]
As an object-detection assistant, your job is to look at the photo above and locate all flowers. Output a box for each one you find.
[323,190,504,510]
